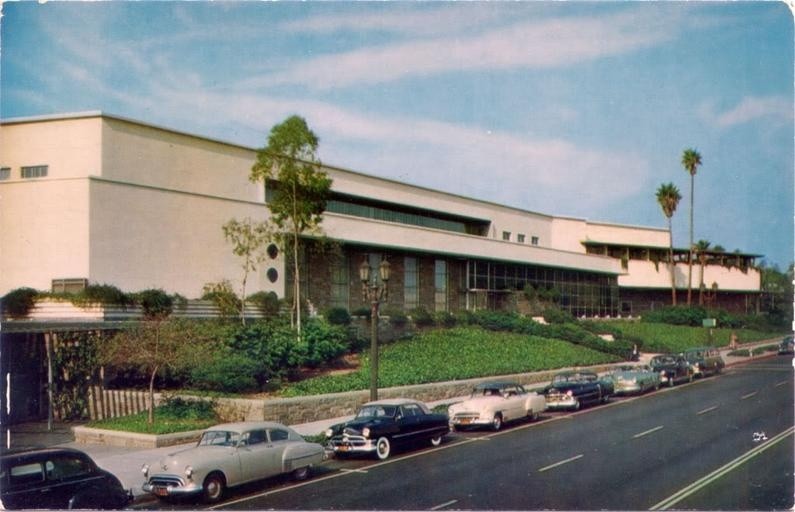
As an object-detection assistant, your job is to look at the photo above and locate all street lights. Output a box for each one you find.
[700,283,718,344]
[360,256,391,402]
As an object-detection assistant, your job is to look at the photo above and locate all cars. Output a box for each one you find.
[326,397,452,461]
[613,364,661,394]
[685,346,725,377]
[141,420,328,503]
[651,353,695,387]
[447,380,545,431]
[778,334,795,355]
[0,448,134,509]
[537,368,615,410]
